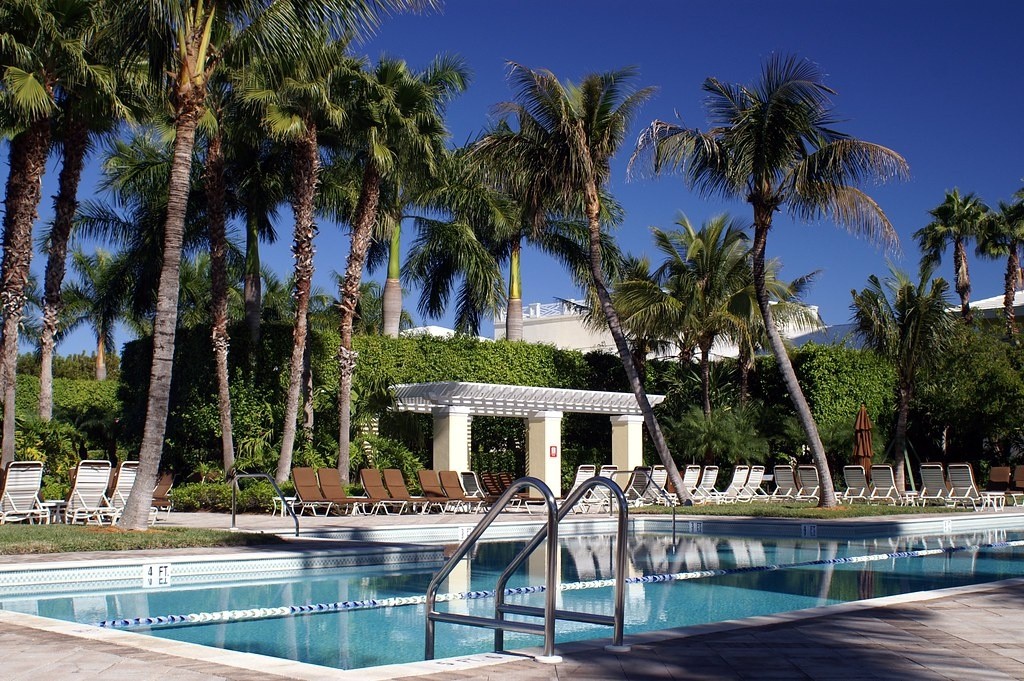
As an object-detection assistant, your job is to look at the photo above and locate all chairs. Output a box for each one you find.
[271,467,521,517]
[497,471,563,514]
[0,460,175,526]
[525,463,1024,514]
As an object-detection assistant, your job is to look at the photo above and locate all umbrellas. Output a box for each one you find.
[852,405,874,486]
